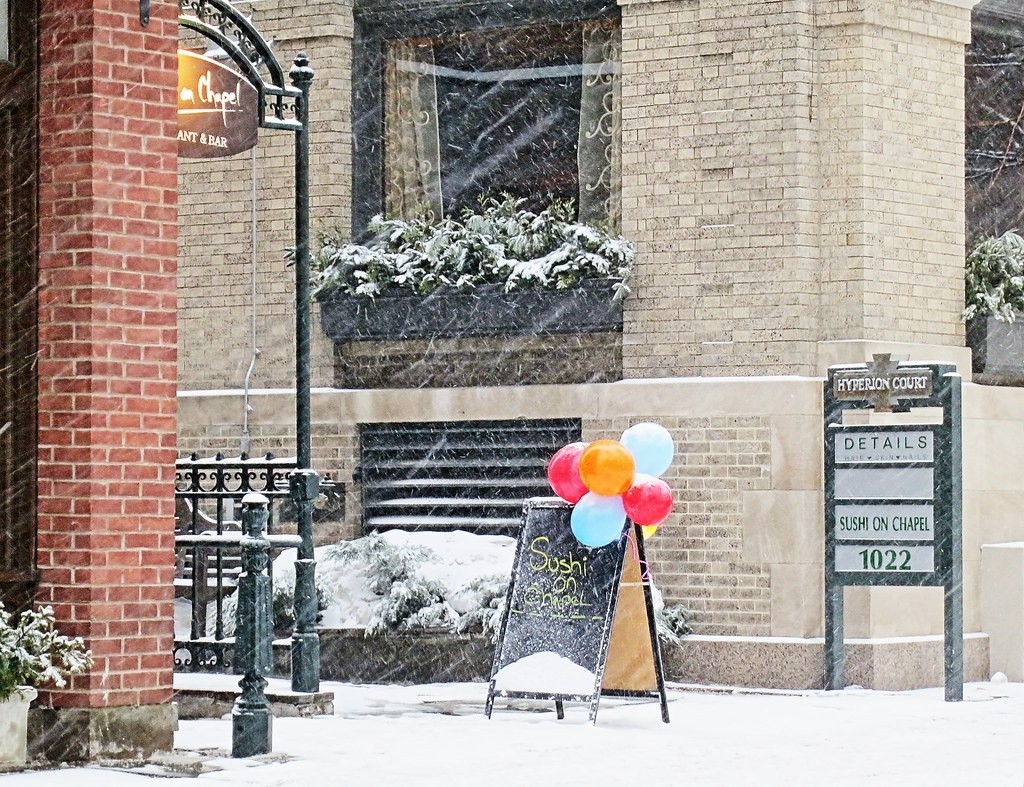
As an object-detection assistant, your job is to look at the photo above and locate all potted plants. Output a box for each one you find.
[0,601,95,774]
[284,190,640,340]
[966,226,1024,387]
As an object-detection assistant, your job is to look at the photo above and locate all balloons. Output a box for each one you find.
[548,423,674,547]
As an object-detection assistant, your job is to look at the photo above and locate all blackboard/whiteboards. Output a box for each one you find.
[489,499,632,702]
